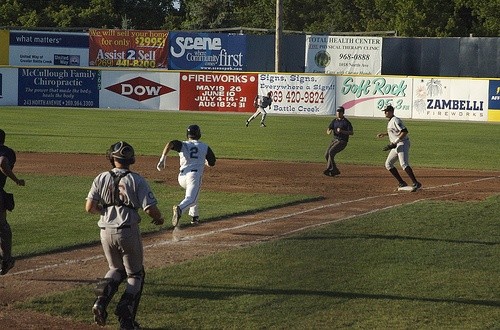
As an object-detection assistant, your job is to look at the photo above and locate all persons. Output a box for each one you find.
[246,92,272,127]
[85,140,165,330]
[157,125,216,226]
[324,107,353,176]
[377,105,422,192]
[0,129,25,275]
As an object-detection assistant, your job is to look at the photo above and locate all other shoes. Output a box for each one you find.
[325,168,341,176]
[260,122,266,127]
[245,121,249,127]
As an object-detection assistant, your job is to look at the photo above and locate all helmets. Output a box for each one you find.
[186,125,201,138]
[107,142,135,164]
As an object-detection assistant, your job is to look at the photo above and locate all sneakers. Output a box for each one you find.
[121,318,139,329]
[191,218,200,225]
[91,299,109,327]
[398,182,407,187]
[411,182,422,192]
[172,205,182,226]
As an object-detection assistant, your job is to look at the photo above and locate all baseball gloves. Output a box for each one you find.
[382,144,397,151]
[5,193,15,212]
[253,103,259,108]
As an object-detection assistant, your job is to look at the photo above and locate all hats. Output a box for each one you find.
[382,105,394,111]
[336,106,345,112]
